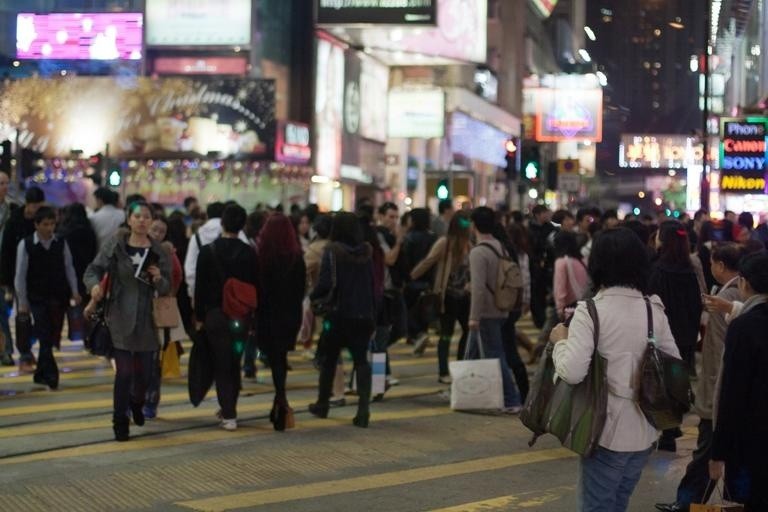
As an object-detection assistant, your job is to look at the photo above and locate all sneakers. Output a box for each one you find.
[353,408,370,426]
[330,377,399,406]
[1,355,57,389]
[114,400,157,441]
[270,400,292,431]
[309,401,328,418]
[221,417,237,430]
[655,429,682,452]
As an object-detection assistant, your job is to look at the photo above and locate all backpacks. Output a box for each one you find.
[475,242,521,312]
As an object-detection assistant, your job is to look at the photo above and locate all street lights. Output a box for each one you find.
[668,16,709,215]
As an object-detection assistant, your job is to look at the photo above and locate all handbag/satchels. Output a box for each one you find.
[519,340,607,459]
[352,351,387,395]
[222,278,258,319]
[89,324,112,356]
[84,271,112,323]
[159,342,180,379]
[640,342,692,431]
[449,359,505,411]
[189,338,214,405]
[153,296,179,328]
[417,292,441,321]
[311,286,343,316]
[690,502,744,512]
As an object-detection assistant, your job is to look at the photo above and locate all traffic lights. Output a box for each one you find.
[108,163,122,187]
[503,136,518,181]
[436,178,450,200]
[0,139,12,176]
[521,139,540,181]
[21,149,42,179]
[87,153,103,187]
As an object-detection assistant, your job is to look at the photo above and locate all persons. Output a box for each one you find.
[163,196,557,431]
[0,174,125,391]
[550,204,768,451]
[707,249,768,509]
[546,225,682,511]
[84,194,173,440]
[654,240,745,509]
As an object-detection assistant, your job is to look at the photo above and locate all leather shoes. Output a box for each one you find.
[654,502,683,512]
